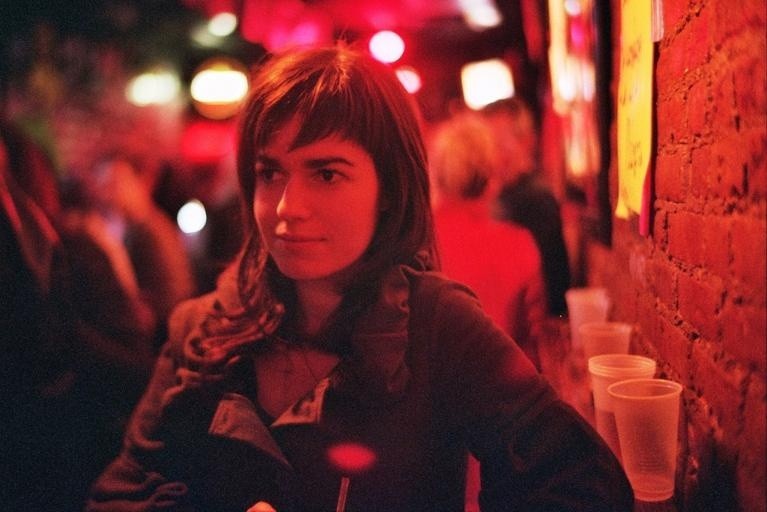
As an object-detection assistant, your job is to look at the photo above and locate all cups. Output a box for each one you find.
[565,285,685,502]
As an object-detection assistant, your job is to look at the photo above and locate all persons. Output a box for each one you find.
[0,98,572,512]
[77,43,635,510]
